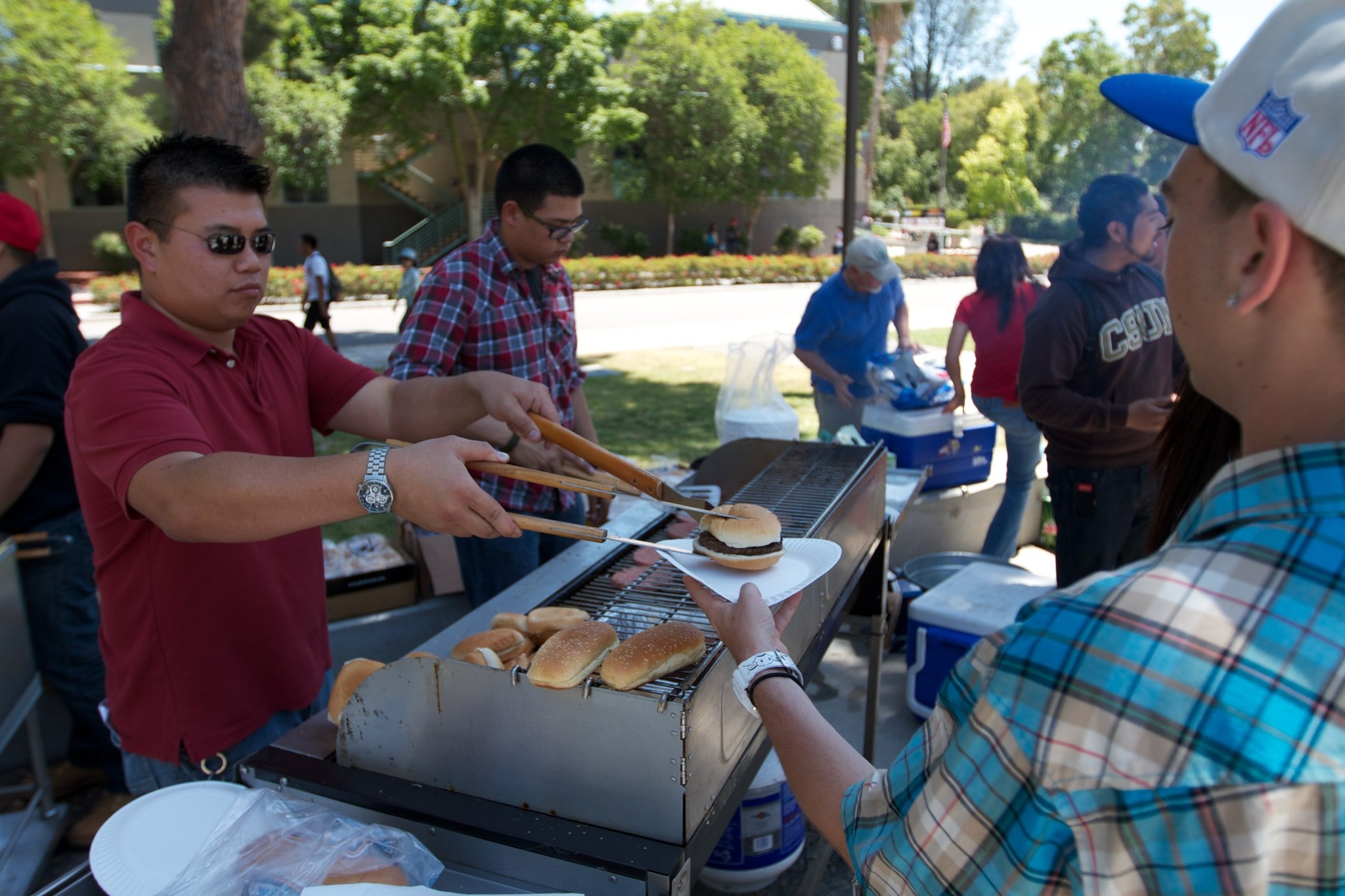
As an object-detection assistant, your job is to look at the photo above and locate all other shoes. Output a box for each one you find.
[21,756,112,801]
[68,789,137,848]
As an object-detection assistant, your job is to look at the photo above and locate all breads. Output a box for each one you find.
[449,606,708,690]
[326,651,443,728]
[233,831,408,894]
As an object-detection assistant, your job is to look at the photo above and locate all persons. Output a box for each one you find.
[683,0,1345,896]
[380,141,611,611]
[0,196,137,846]
[1018,173,1241,587]
[833,227,843,255]
[793,235,920,442]
[62,128,560,811]
[297,233,338,353]
[702,224,719,256]
[862,210,884,230]
[942,233,1049,558]
[393,248,421,334]
[726,218,742,254]
[927,234,939,254]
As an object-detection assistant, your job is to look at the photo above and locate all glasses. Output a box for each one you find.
[514,202,589,240]
[142,218,277,254]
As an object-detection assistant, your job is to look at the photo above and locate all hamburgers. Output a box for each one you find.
[693,502,786,570]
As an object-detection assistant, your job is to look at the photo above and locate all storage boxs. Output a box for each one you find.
[861,401,998,490]
[902,562,1057,721]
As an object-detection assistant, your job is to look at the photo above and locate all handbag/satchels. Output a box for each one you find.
[328,268,344,301]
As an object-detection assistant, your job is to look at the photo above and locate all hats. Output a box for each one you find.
[0,194,42,253]
[398,248,417,262]
[1100,0,1345,255]
[845,236,901,283]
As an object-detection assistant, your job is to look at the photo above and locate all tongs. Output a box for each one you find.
[383,439,741,562]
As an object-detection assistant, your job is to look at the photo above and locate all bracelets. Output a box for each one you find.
[748,672,805,709]
[505,434,521,453]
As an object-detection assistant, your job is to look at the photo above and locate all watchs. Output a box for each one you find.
[731,649,812,720]
[356,446,393,516]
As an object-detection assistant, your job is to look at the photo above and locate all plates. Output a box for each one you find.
[721,409,798,443]
[88,782,248,896]
[653,538,842,608]
